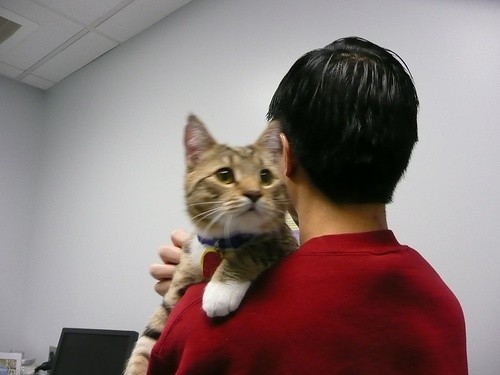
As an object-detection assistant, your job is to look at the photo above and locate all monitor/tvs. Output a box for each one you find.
[52,327,139,375]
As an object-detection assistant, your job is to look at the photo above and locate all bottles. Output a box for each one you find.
[21,357,39,375]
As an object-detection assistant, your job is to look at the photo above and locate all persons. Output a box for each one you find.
[145,36,467,375]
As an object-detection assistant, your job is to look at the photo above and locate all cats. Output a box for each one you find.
[124,113,299,375]
